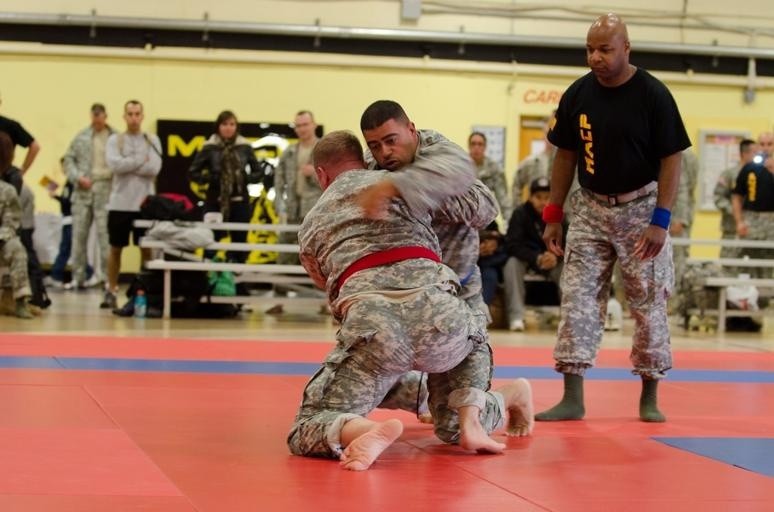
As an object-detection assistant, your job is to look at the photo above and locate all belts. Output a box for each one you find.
[595,181,657,206]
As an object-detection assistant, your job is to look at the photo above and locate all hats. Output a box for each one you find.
[531,176,551,194]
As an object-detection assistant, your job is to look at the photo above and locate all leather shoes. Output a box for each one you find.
[101,293,116,307]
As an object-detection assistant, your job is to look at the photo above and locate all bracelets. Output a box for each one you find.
[649,206,671,230]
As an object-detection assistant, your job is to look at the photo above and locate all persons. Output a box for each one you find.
[469,131,510,221]
[267,109,331,315]
[478,221,506,303]
[510,126,578,216]
[0,179,33,318]
[668,152,697,316]
[714,131,774,309]
[47,157,98,289]
[285,129,509,473]
[18,185,36,237]
[64,103,119,292]
[190,112,265,296]
[0,115,40,194]
[502,176,569,331]
[533,10,693,423]
[100,100,162,310]
[353,98,535,445]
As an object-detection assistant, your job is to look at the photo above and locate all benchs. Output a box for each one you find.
[497,221,547,282]
[670,237,774,333]
[134,219,315,319]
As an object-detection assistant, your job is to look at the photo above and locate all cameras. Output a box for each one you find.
[752,152,767,172]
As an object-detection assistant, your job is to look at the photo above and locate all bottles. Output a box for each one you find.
[133,289,147,318]
[252,304,265,321]
[524,309,538,334]
